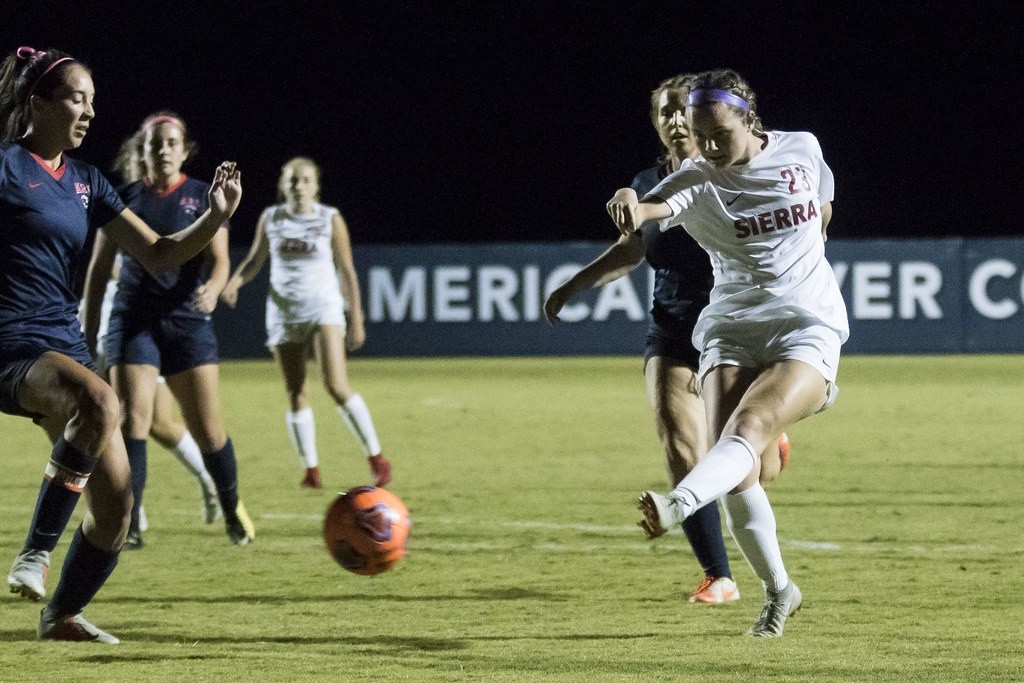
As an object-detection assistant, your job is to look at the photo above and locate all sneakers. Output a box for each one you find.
[366,452,392,487]
[300,466,322,487]
[36,606,120,645]
[749,578,803,638]
[7,548,50,602]
[138,505,147,532]
[689,576,740,604]
[201,490,223,524]
[121,531,145,550]
[637,490,691,539]
[223,496,255,548]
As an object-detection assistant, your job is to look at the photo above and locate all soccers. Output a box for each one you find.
[321,483,410,577]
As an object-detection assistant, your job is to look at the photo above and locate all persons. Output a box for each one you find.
[221,158,392,487]
[606,71,851,638]
[545,73,791,603]
[0,44,242,644]
[76,112,255,547]
